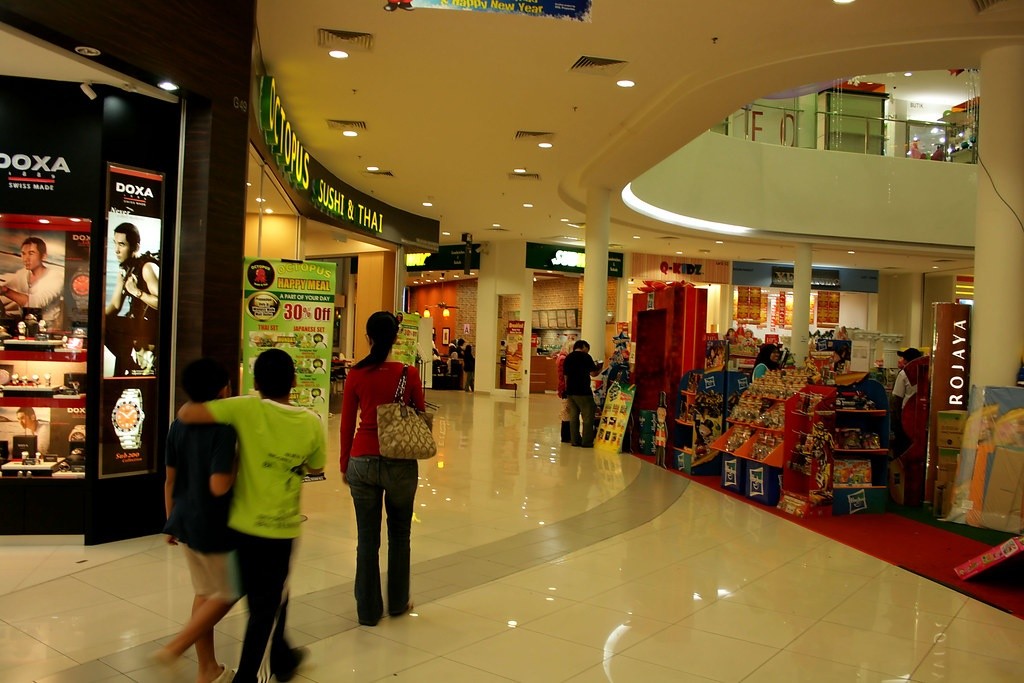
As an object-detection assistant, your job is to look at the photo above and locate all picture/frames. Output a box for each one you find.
[442,327,450,346]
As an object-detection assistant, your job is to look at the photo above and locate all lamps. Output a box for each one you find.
[442,273,450,317]
[415,287,420,315]
[605,315,614,324]
[423,283,431,317]
[80,80,97,100]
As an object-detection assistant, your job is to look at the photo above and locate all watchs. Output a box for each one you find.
[18,320,46,334]
[12,374,51,386]
[22,452,42,459]
[111,388,144,450]
[69,425,86,455]
[70,266,89,314]
[136,290,143,298]
[62,336,69,348]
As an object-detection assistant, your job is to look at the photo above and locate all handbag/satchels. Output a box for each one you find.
[376,364,437,460]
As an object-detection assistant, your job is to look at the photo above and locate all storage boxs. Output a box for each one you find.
[936,408,969,518]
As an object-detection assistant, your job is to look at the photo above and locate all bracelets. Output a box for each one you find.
[3,289,10,296]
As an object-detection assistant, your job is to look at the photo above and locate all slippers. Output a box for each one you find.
[210,663,240,683]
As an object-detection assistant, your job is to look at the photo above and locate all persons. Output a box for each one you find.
[0,237,64,326]
[149,357,244,683]
[890,348,922,456]
[557,340,601,447]
[751,344,779,384]
[726,329,756,348]
[179,349,326,683]
[104,223,159,376]
[17,407,51,453]
[340,311,426,627]
[432,334,476,392]
[833,344,851,371]
[710,343,725,366]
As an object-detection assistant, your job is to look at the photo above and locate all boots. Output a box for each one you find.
[561,420,572,443]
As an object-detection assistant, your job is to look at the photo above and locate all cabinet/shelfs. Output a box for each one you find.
[937,106,979,163]
[0,345,88,409]
[668,339,888,521]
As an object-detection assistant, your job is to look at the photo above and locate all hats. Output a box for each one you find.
[897,348,921,362]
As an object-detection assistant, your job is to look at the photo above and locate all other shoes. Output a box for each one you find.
[390,603,413,616]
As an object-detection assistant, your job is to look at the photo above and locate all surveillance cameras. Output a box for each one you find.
[80,84,97,100]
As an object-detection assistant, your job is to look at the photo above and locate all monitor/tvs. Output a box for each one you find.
[817,339,852,362]
